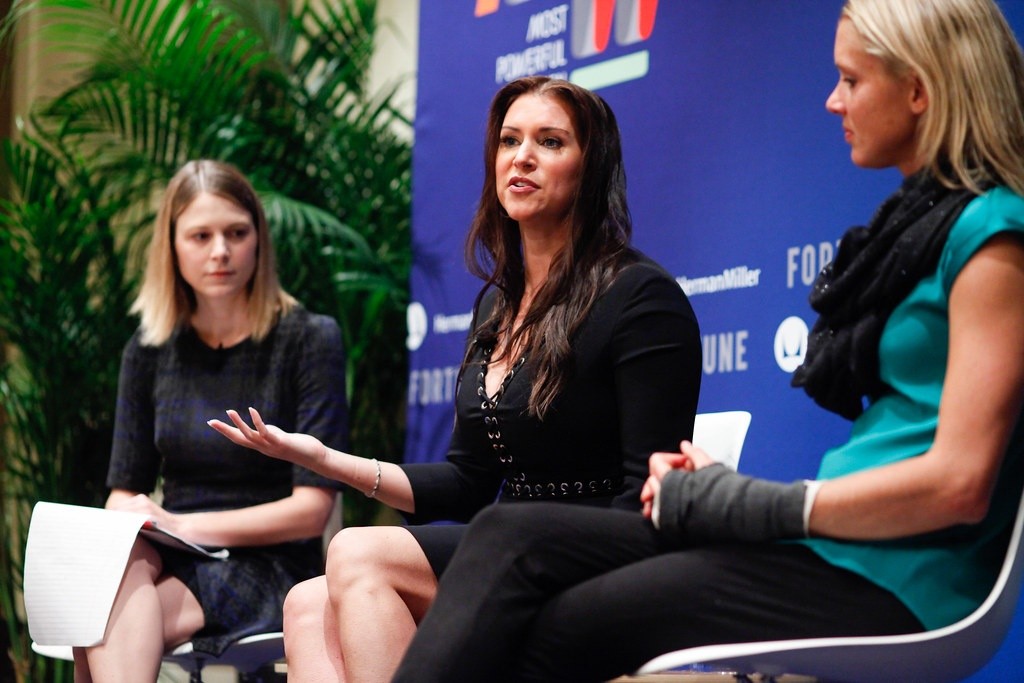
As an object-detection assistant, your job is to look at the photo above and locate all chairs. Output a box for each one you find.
[637,492,1024,683]
[31,628,286,683]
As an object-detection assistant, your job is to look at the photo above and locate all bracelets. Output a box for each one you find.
[365,457,381,500]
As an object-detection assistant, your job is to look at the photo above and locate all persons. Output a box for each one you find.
[206,75,704,683]
[388,0,1023,683]
[72,156,352,683]
